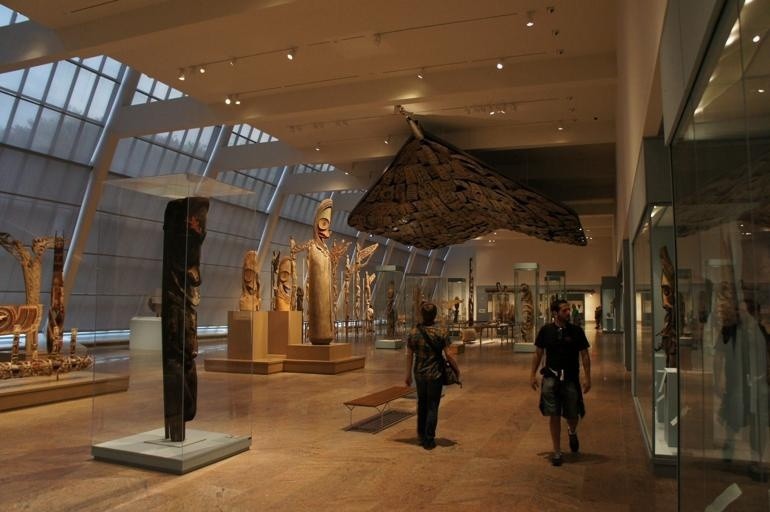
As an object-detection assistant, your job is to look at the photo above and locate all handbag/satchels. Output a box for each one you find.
[419,324,460,385]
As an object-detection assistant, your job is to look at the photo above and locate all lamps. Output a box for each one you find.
[314,142,322,151]
[417,66,427,80]
[495,58,505,69]
[463,102,518,117]
[383,134,393,145]
[554,96,577,132]
[224,93,241,106]
[520,6,566,58]
[175,54,235,83]
[288,118,350,134]
[286,48,297,61]
[371,32,383,46]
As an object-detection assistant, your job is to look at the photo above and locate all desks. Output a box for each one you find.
[480,323,515,346]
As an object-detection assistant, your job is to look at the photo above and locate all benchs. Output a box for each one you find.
[451,343,466,355]
[343,385,416,430]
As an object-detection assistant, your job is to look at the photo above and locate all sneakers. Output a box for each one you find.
[567,426,580,454]
[552,451,562,466]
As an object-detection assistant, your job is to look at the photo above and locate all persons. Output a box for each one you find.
[598,306,602,327]
[405,299,464,451]
[709,297,769,482]
[595,307,600,329]
[528,296,594,466]
[572,301,581,324]
[577,304,583,316]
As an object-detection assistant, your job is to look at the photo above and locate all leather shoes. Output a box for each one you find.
[425,438,436,450]
